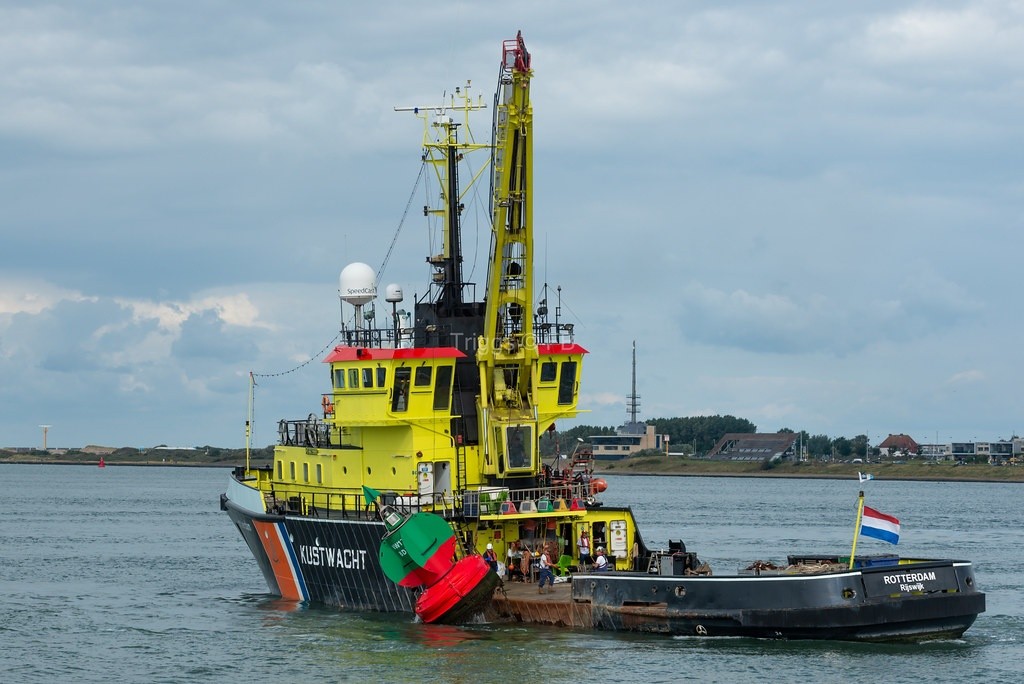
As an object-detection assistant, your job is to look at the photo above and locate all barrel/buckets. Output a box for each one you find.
[289,497,299,509]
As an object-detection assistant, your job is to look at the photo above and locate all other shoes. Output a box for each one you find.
[548,584,556,592]
[539,587,547,594]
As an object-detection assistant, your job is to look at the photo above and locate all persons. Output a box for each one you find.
[507,540,524,583]
[482,543,498,573]
[592,546,608,571]
[576,530,590,570]
[512,437,526,467]
[538,545,558,594]
[539,463,546,488]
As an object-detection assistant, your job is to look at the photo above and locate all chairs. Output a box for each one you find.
[553,555,572,577]
[479,491,508,515]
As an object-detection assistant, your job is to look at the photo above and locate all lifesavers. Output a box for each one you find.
[322,396,330,414]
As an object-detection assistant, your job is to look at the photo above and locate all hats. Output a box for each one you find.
[582,530,588,533]
[596,546,604,552]
[486,543,492,549]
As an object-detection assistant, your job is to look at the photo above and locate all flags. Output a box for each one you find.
[859,504,900,545]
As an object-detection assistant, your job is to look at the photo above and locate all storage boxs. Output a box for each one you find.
[787,554,900,568]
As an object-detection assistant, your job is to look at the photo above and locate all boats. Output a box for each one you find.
[217,28,988,647]
